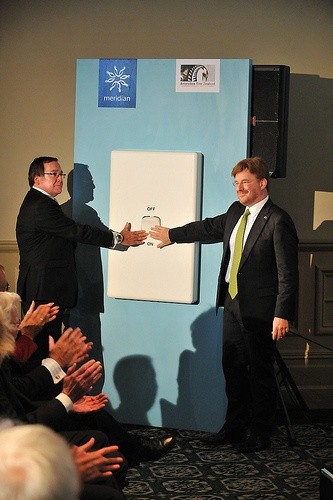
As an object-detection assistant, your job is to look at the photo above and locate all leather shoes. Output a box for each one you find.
[200,429,247,445]
[126,436,176,466]
[232,433,271,452]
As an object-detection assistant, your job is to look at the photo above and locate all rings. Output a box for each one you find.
[40,318,43,321]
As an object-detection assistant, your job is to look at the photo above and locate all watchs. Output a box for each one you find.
[116,231,124,245]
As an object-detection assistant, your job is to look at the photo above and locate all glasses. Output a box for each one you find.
[44,172,66,180]
[232,177,258,187]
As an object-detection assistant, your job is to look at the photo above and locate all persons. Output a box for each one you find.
[151,155,301,454]
[0,264,12,292]
[0,291,176,500]
[14,155,149,356]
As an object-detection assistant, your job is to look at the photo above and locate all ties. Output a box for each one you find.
[228,208,251,300]
